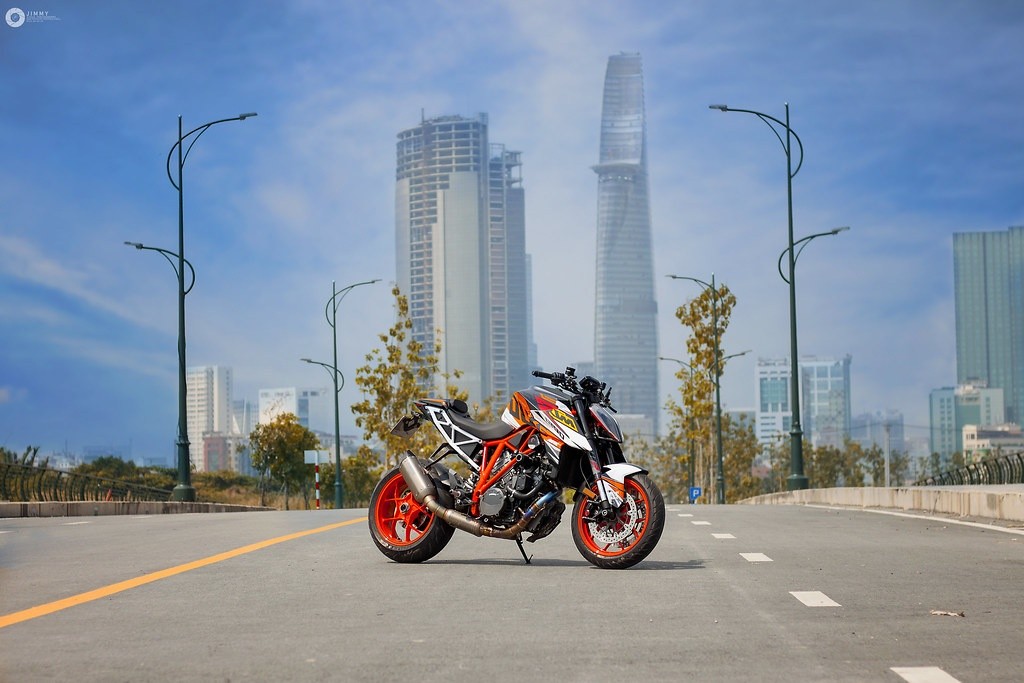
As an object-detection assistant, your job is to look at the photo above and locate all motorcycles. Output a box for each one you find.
[368,366,665,570]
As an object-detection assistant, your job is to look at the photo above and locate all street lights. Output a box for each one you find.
[299,278,385,511]
[660,273,755,505]
[124,111,259,502]
[709,101,851,489]
